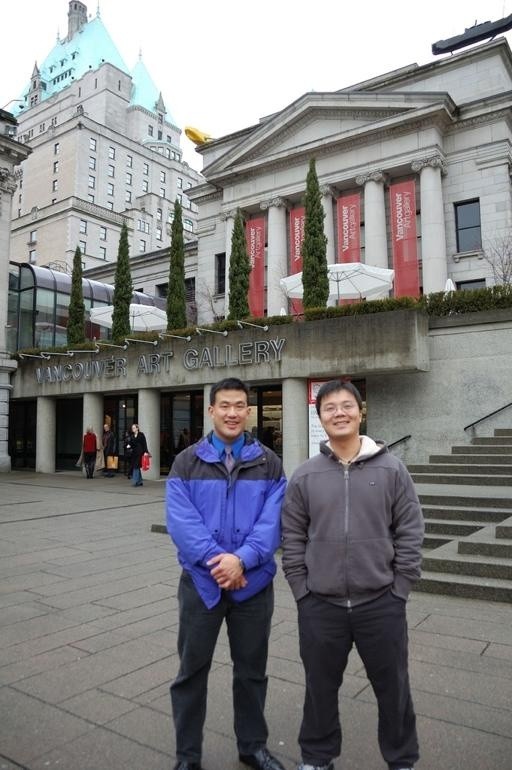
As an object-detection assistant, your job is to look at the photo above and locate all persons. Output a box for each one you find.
[278,379,426,769]
[165,376,294,770]
[125,423,149,486]
[122,429,133,476]
[102,423,116,477]
[178,427,191,452]
[81,425,101,479]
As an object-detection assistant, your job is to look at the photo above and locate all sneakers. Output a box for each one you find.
[102,473,143,487]
[296,759,336,770]
[387,763,416,770]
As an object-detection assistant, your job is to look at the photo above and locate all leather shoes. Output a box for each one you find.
[172,758,203,770]
[238,744,286,770]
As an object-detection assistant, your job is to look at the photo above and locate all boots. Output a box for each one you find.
[84,461,94,479]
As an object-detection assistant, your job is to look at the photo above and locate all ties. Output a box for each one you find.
[221,445,237,473]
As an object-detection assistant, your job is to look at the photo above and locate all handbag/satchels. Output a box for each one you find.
[141,454,151,471]
[106,456,120,469]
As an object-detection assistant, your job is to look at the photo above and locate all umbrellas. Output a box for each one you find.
[278,260,396,306]
[87,305,174,333]
[442,278,457,302]
